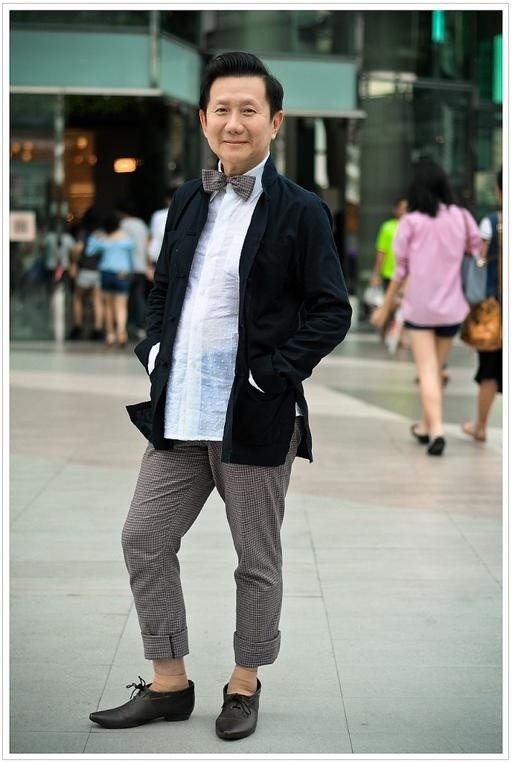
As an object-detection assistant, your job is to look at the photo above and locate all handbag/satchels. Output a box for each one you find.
[459,295,502,351]
[465,246,487,303]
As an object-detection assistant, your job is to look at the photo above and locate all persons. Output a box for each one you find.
[89,54,351,740]
[46,186,178,347]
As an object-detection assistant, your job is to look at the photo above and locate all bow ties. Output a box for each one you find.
[200,164,255,206]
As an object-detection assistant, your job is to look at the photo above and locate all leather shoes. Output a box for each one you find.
[216,676,262,743]
[425,435,445,456]
[463,420,485,441]
[411,420,428,444]
[88,676,195,727]
